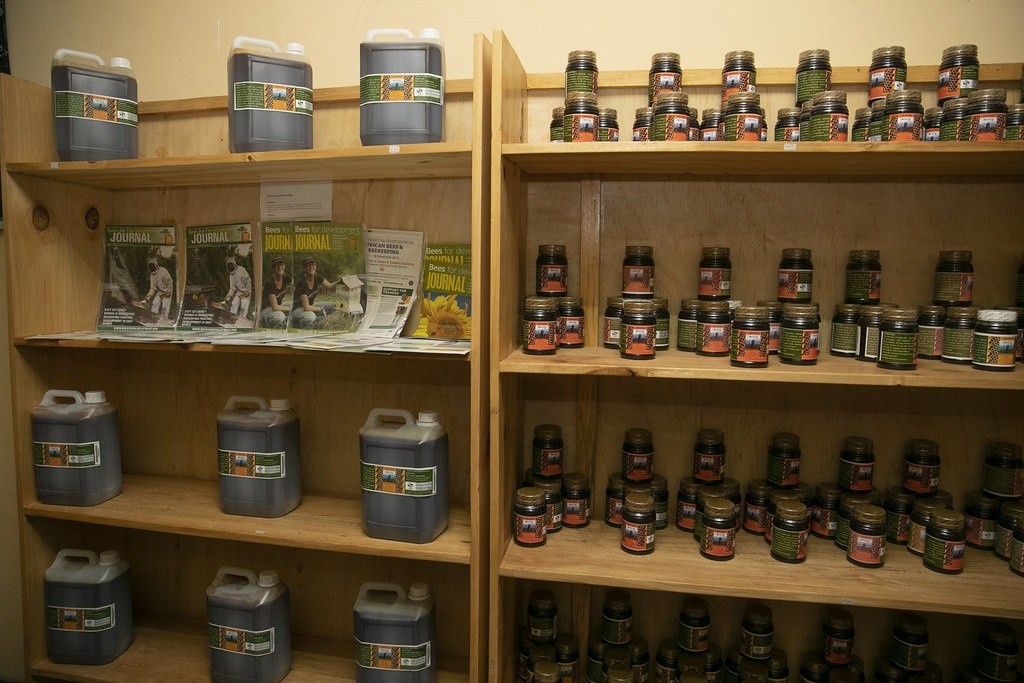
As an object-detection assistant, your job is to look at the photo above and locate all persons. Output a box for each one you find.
[144,258,173,318]
[223,258,251,317]
[292,256,336,329]
[261,257,294,329]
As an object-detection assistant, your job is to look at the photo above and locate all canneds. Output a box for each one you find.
[549,43,1024,140]
[515,423,1023,575]
[521,244,1024,372]
[514,590,1024,683]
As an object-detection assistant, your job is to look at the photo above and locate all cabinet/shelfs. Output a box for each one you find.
[0,28,1023,683]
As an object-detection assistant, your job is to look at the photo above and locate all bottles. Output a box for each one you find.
[359,29,444,146]
[51,49,137,160]
[30,390,123,508]
[352,581,437,683]
[214,394,302,519]
[359,407,449,544]
[206,566,292,683]
[44,549,132,665]
[227,36,315,154]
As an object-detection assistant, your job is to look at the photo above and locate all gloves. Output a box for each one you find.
[336,278,345,284]
[163,292,171,297]
[244,290,250,298]
[321,307,326,319]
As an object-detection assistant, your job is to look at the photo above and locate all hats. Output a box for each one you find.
[302,255,314,267]
[272,256,286,267]
